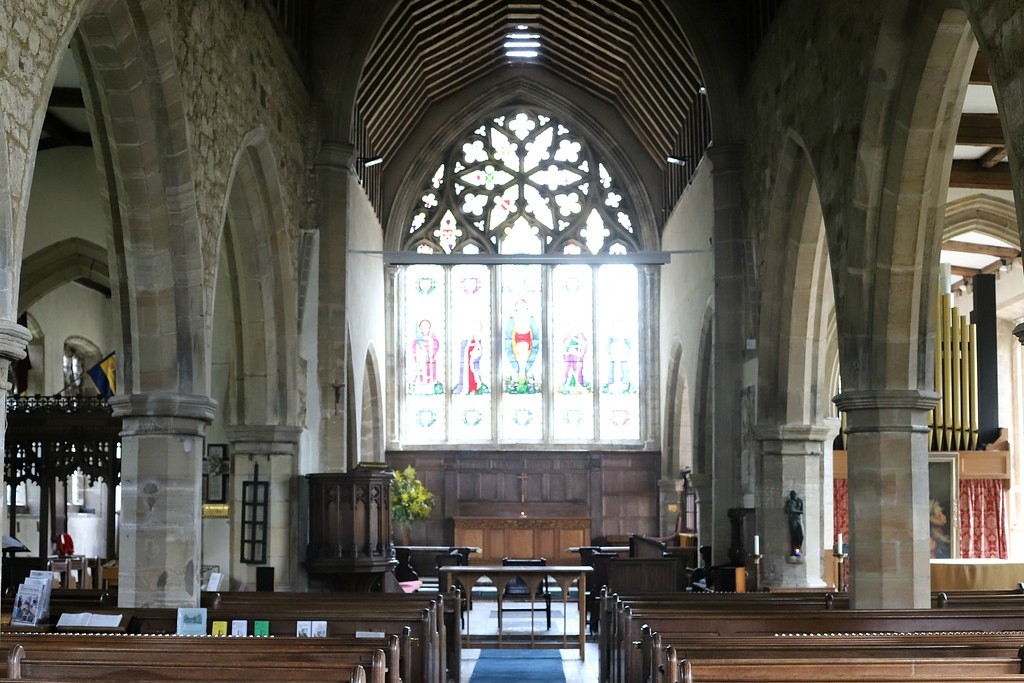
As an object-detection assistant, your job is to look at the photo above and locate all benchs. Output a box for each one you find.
[0,555,466,683]
[600,583,1024,683]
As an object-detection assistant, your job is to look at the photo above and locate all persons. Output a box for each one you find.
[785,490,805,557]
[21,602,35,622]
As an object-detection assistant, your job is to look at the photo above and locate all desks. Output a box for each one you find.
[567,547,668,613]
[930,558,1024,591]
[452,516,592,566]
[440,566,594,662]
[394,546,482,610]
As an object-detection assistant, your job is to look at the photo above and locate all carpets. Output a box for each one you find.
[469,649,566,683]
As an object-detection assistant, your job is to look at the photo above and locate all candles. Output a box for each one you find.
[838,533,843,554]
[754,535,759,555]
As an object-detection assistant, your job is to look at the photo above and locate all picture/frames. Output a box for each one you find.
[928,452,960,559]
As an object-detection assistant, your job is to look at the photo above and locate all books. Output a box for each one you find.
[232,620,247,637]
[297,621,312,637]
[177,607,207,635]
[355,631,385,638]
[11,570,54,626]
[212,621,227,636]
[2,536,23,548]
[311,621,327,637]
[254,621,269,638]
[56,612,123,628]
[206,572,224,592]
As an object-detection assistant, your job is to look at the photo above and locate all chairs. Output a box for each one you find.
[498,557,551,631]
